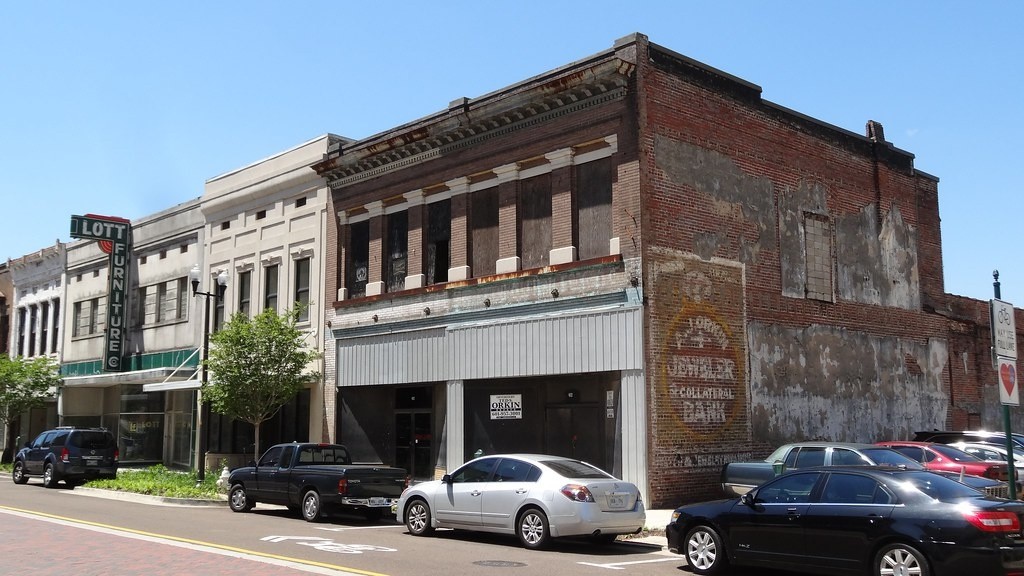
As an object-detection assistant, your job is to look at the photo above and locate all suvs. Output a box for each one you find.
[12,426,120,488]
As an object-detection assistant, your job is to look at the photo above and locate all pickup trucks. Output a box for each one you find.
[228,443,409,526]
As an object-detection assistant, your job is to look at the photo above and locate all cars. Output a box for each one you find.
[396,455,647,550]
[666,464,1024,576]
[873,431,1023,501]
[722,442,1009,500]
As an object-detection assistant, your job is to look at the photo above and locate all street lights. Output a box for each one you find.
[190,263,230,486]
